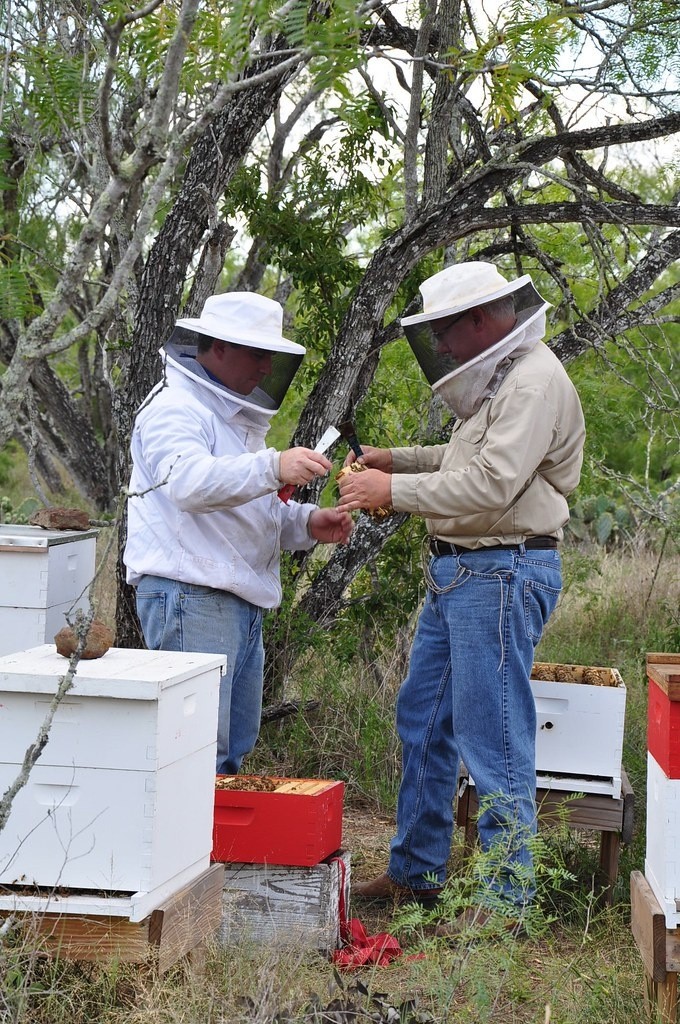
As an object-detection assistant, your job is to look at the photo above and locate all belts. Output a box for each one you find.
[429,536,557,557]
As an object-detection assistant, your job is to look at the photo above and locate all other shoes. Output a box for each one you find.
[437,905,525,945]
[351,872,445,902]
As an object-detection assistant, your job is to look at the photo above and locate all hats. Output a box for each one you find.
[157,291,308,415]
[400,262,553,419]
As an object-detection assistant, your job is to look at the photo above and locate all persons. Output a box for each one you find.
[335,261,586,947]
[122,291,355,776]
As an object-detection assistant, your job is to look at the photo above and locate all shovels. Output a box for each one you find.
[277,425,342,507]
[339,420,363,458]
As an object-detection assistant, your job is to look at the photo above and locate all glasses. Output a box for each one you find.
[433,310,470,341]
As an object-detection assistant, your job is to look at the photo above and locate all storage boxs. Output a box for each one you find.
[629,870,680,985]
[210,773,344,866]
[0,741,218,892]
[465,660,628,801]
[456,762,636,845]
[0,596,91,658]
[0,536,96,609]
[0,665,221,771]
[0,862,224,978]
[643,750,680,911]
[210,850,352,952]
[647,677,680,781]
